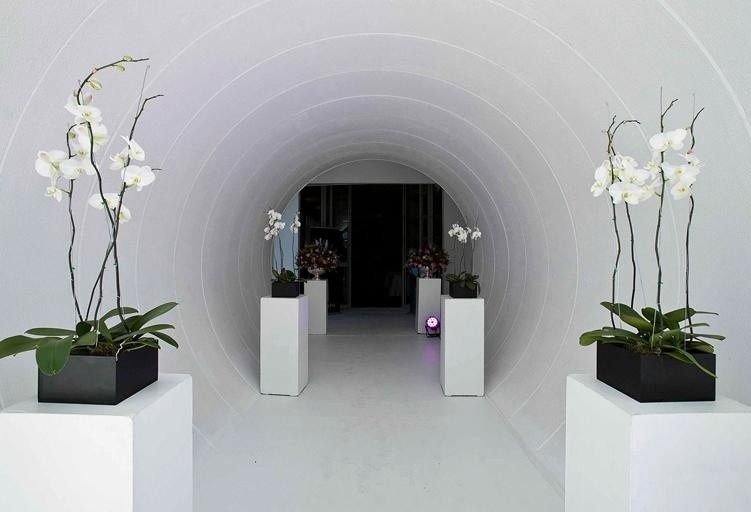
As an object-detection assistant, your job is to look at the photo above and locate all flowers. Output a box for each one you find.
[0,56,179,377]
[404,243,450,271]
[441,217,481,296]
[294,237,340,273]
[264,208,307,283]
[579,99,725,377]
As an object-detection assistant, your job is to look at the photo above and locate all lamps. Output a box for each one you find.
[426,316,440,338]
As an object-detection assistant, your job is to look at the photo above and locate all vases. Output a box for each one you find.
[597,340,716,403]
[450,282,477,298]
[308,268,325,280]
[420,267,434,278]
[38,339,158,405]
[272,282,299,298]
